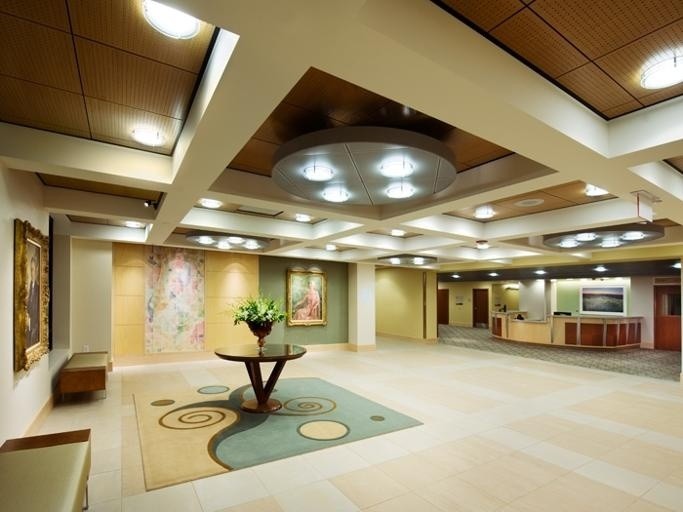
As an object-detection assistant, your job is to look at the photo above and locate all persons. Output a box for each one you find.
[293,280,320,320]
[26,256,39,345]
[517,314,524,320]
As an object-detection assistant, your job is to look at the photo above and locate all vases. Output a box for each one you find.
[248,319,275,355]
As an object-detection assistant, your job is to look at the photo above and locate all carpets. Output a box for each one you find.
[132,374,424,492]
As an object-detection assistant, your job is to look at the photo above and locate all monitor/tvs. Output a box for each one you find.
[554,312,572,316]
[504,304,506,312]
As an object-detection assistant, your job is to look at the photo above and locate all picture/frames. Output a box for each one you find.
[13,217,52,370]
[285,269,328,327]
[579,285,628,317]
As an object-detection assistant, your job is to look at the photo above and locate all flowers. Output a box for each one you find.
[226,290,290,326]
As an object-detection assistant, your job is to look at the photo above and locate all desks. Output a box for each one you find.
[214,342,308,417]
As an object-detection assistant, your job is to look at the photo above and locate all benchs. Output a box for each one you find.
[58,351,109,402]
[0,426,94,512]
